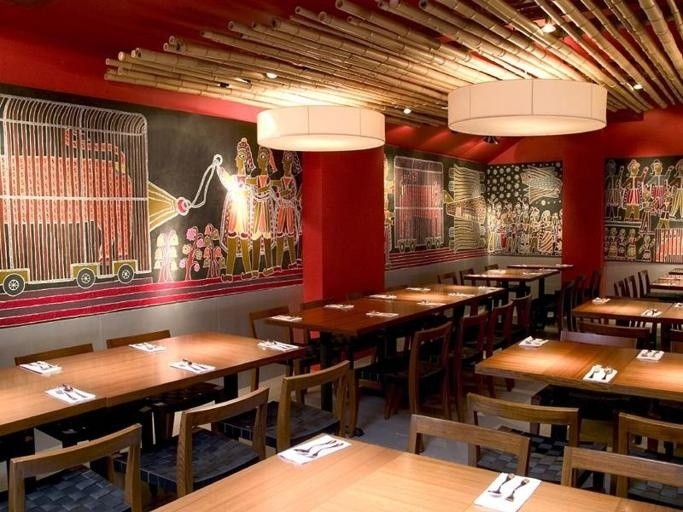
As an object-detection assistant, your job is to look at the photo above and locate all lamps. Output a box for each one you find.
[441,73,611,140]
[248,93,391,155]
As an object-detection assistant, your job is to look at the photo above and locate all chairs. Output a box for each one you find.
[1,330,356,512]
[467,267,683,511]
[247,264,585,438]
[410,414,530,478]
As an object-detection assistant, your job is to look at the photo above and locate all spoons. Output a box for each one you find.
[62,384,86,399]
[292,439,335,455]
[484,473,515,497]
[181,358,207,370]
[600,364,613,379]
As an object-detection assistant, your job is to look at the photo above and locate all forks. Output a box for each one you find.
[306,442,343,458]
[587,365,601,379]
[505,478,530,502]
